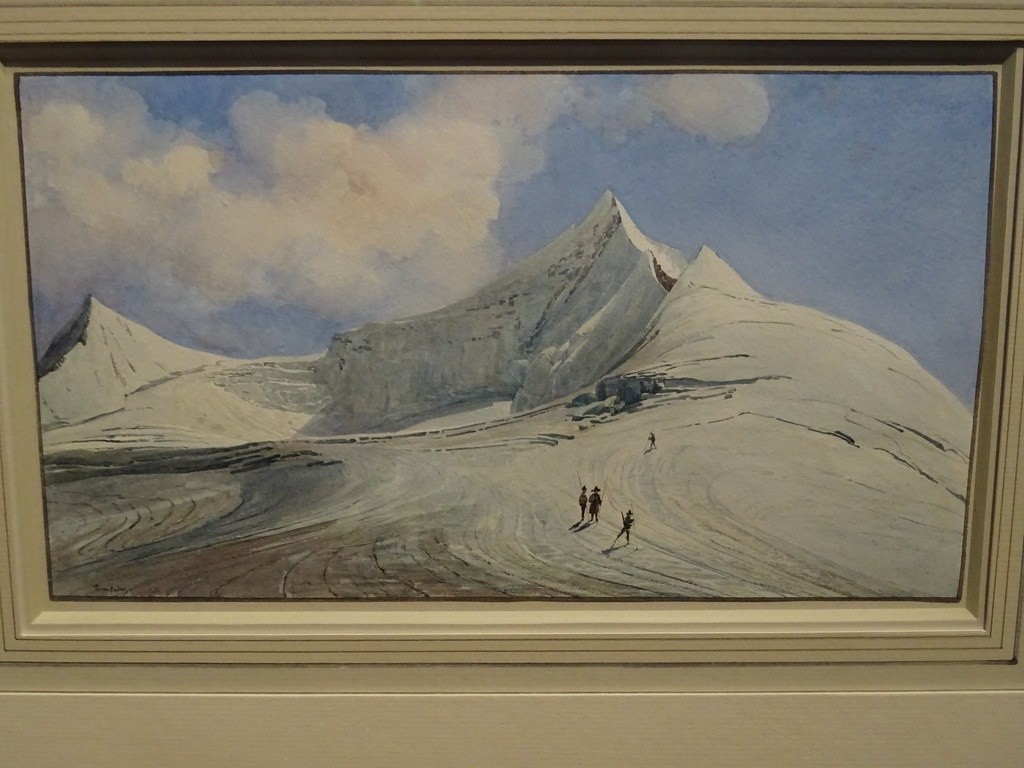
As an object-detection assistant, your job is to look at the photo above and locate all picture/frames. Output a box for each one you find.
[0,0,1024,670]
